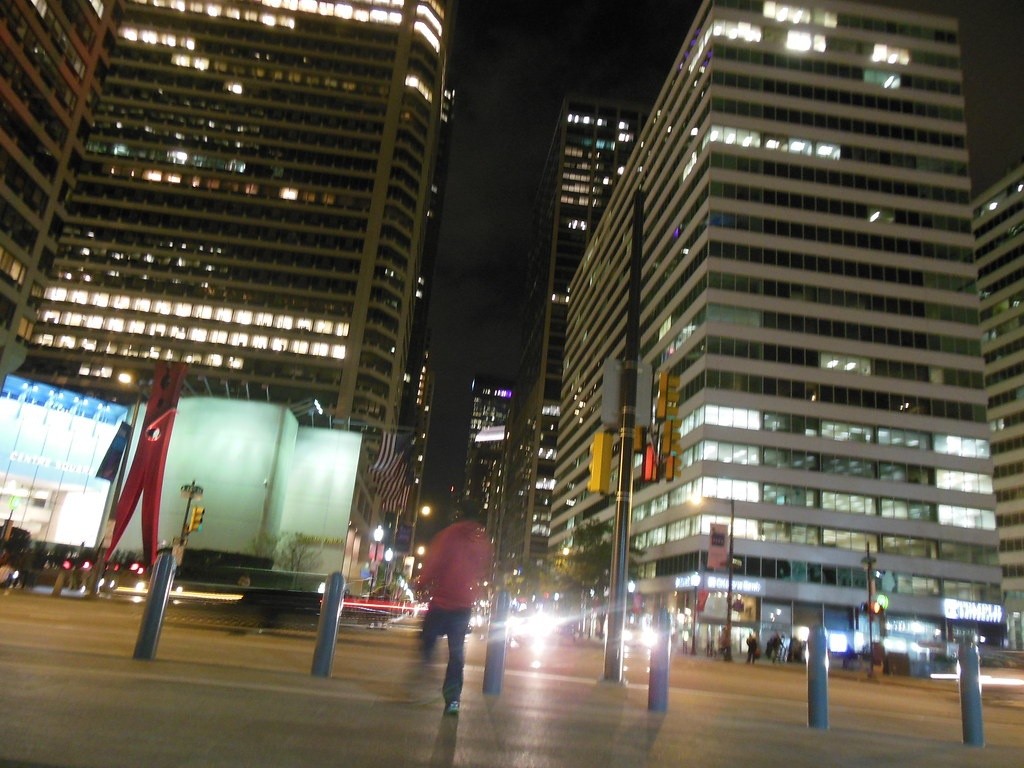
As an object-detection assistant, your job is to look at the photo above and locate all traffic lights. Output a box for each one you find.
[658,368,681,421]
[585,431,614,497]
[871,601,882,613]
[188,508,205,535]
[662,419,683,457]
[666,453,683,484]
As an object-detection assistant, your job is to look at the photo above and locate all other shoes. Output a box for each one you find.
[444,700,460,715]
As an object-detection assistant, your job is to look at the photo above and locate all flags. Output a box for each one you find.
[367,425,419,514]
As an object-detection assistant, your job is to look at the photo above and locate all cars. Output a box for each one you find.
[0,526,32,587]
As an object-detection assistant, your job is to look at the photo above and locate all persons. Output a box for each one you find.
[843,644,859,671]
[412,499,494,714]
[746,633,806,665]
[0,548,34,589]
